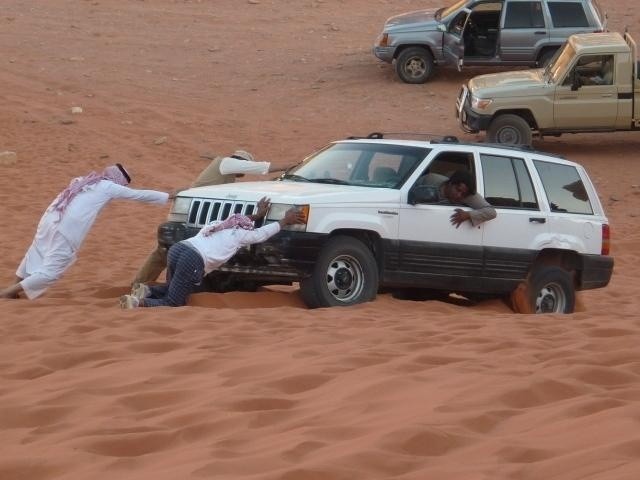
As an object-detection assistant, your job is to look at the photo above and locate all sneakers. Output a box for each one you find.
[119,295,140,310]
[130,283,152,299]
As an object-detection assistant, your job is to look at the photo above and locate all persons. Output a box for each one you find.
[119,194,306,310]
[0,162,190,302]
[127,149,304,295]
[408,170,498,230]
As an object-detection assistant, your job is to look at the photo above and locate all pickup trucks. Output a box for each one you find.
[454,31,640,146]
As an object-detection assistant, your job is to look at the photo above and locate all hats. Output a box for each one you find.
[233,150,253,161]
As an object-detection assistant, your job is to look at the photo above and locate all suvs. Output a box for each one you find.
[373,0,604,84]
[156,132,615,314]
